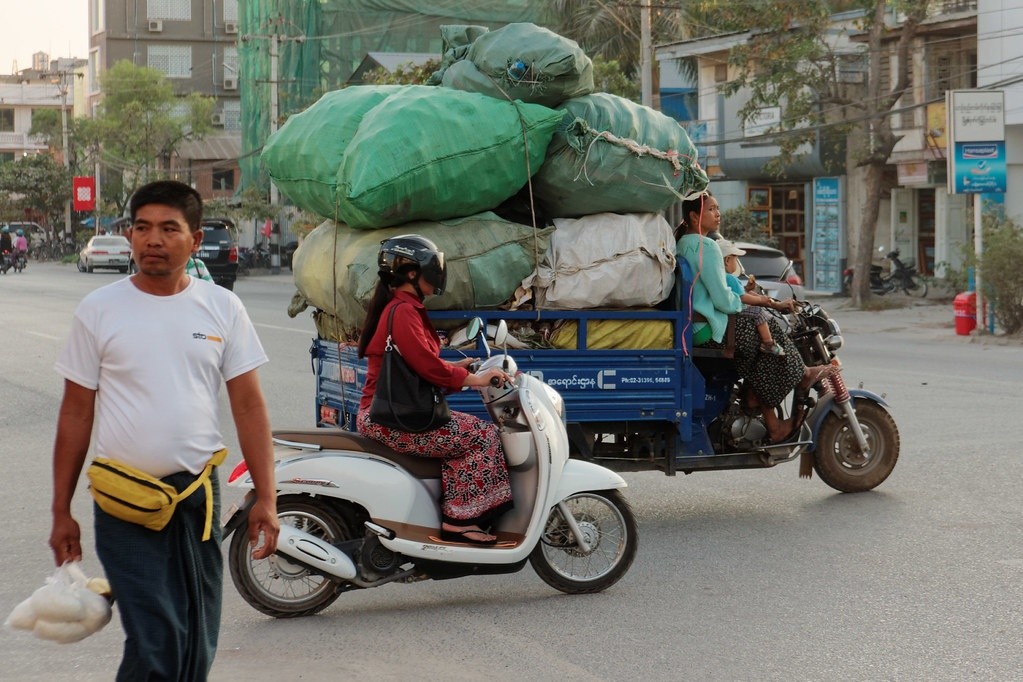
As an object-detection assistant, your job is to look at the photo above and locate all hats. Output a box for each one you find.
[716,239,747,258]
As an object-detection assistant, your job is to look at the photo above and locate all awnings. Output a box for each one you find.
[79,216,128,230]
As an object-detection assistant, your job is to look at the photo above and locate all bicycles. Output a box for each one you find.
[31,238,78,262]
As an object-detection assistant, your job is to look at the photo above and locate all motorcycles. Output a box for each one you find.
[843,247,929,299]
[217,316,640,618]
[0,249,28,274]
[308,260,901,493]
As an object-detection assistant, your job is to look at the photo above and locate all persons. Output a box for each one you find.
[356,234,515,548]
[0,225,27,274]
[672,190,809,445]
[716,240,784,357]
[184,246,263,285]
[48,179,280,682]
[101,224,133,242]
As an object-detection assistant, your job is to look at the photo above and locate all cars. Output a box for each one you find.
[76,235,132,274]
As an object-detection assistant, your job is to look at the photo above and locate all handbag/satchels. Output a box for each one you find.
[369,301,452,432]
[5,556,116,644]
[84,458,177,531]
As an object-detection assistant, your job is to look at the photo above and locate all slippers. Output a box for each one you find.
[440,523,498,546]
[805,364,844,390]
[773,407,810,446]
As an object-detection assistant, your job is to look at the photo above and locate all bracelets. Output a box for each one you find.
[767,297,774,308]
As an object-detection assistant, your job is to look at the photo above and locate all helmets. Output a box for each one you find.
[17,229,23,236]
[2,226,9,234]
[378,234,447,296]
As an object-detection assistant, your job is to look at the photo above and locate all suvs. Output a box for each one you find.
[0,221,60,250]
[726,240,806,330]
[129,220,239,293]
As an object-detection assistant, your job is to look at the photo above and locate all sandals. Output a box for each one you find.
[760,339,791,356]
